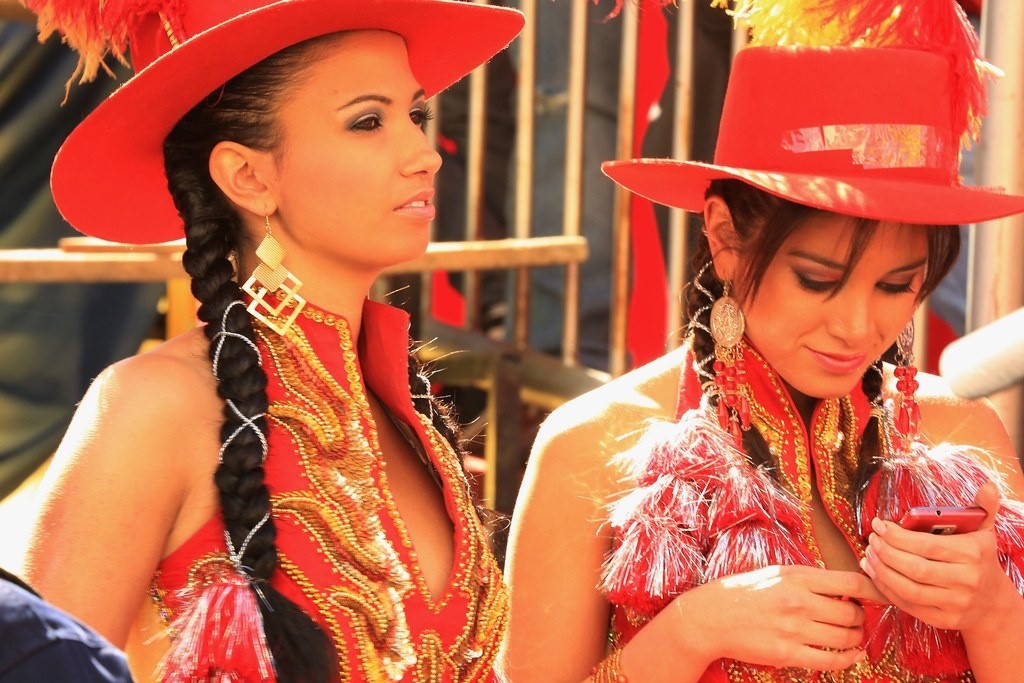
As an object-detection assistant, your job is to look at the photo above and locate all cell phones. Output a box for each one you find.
[840,506,988,607]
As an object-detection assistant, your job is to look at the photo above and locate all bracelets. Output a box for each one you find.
[591,647,628,683]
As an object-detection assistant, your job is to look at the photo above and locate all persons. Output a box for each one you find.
[493,0,1024,683]
[0,1,526,683]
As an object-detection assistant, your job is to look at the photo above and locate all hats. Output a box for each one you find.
[598,44,1024,227]
[46,2,528,253]
